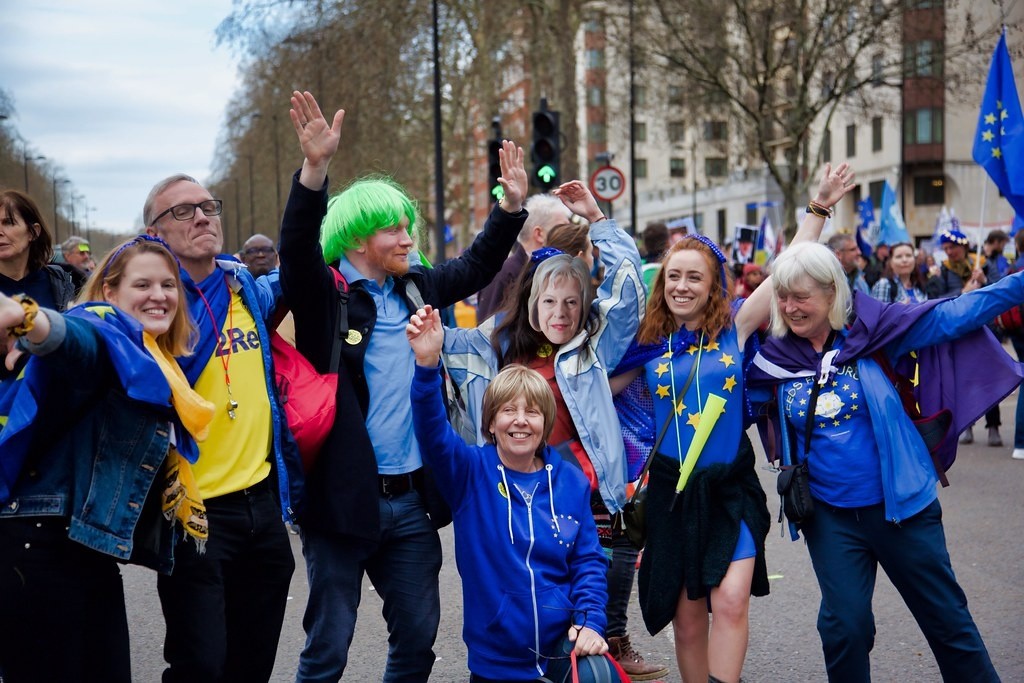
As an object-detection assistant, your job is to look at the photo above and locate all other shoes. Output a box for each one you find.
[1012,448,1024,459]
[989,431,1002,445]
[958,429,973,441]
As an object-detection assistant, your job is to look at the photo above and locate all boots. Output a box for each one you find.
[607,633,670,680]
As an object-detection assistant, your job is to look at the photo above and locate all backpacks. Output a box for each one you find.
[265,266,349,476]
[993,259,1024,331]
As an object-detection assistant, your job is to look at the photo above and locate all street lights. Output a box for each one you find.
[85,205,98,239]
[281,35,324,115]
[53,176,70,246]
[222,177,241,250]
[252,112,281,242]
[234,151,255,235]
[23,154,46,194]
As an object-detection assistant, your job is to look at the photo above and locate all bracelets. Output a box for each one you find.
[806,200,833,219]
[5,293,40,338]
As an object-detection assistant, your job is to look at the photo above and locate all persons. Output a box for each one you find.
[733,227,755,263]
[0,89,672,683]
[603,161,871,682]
[743,240,1024,683]
[722,230,1024,460]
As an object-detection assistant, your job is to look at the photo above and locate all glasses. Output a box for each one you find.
[151,200,223,226]
[245,246,273,255]
[70,251,90,255]
[529,605,586,659]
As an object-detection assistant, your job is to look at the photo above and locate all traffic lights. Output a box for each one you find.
[530,108,561,189]
[486,138,505,203]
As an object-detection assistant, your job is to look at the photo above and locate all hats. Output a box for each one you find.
[743,265,761,274]
[737,228,754,242]
[940,231,969,245]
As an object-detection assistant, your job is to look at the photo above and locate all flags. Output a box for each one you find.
[856,197,877,236]
[878,176,912,246]
[971,30,1024,238]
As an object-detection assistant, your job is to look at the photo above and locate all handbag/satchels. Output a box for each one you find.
[777,466,814,523]
[406,277,476,445]
[613,502,647,551]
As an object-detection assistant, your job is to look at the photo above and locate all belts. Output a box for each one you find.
[379,466,426,494]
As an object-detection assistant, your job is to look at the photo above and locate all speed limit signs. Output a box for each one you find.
[590,165,625,201]
